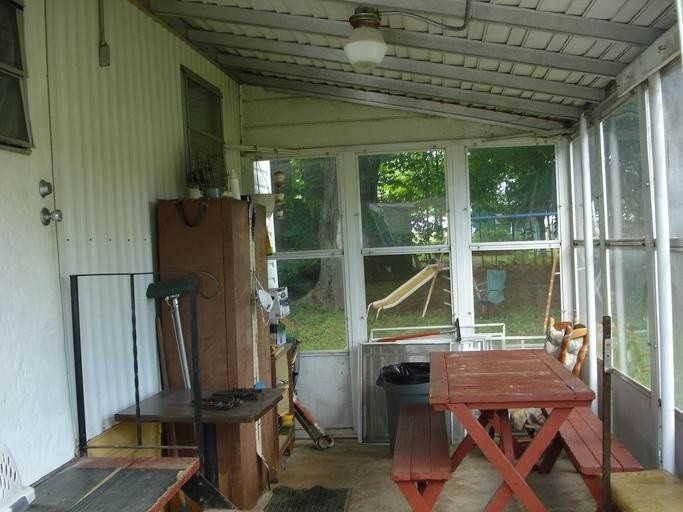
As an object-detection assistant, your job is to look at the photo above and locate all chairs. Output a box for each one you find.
[475,269,508,321]
[497,317,589,451]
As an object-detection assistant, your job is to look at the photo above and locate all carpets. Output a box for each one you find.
[264,485,352,512]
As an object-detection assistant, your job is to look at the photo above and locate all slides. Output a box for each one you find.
[367,265,436,319]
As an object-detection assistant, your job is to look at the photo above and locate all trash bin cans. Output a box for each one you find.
[376,362,430,455]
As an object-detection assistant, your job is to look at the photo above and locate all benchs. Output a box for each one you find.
[391,404,645,512]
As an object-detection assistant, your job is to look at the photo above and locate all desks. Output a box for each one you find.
[16,456,199,511]
[116,388,285,511]
[429,349,596,512]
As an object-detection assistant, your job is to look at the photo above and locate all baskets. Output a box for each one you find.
[249,194,277,218]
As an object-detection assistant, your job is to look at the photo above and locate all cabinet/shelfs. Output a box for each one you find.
[157,199,275,511]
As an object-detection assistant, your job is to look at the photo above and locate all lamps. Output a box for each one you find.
[342,0,471,73]
